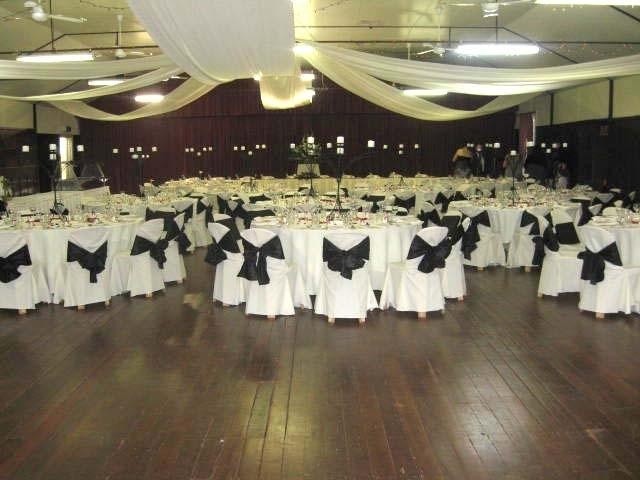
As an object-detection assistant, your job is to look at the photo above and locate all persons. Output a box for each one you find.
[474,143,487,178]
[451,141,474,178]
[502,144,522,178]
[37,155,56,193]
[73,147,108,191]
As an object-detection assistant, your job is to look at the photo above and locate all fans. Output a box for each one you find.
[91,15,153,58]
[448,1,538,15]
[412,7,456,57]
[4,1,87,25]
